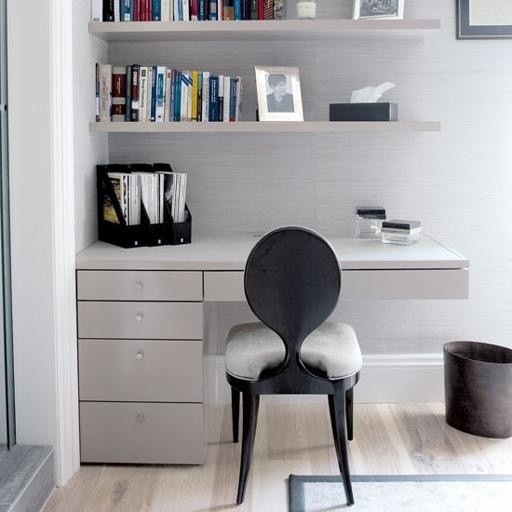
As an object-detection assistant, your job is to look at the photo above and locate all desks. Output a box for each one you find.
[76,230,471,466]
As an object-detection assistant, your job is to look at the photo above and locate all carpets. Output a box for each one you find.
[289,471,511,511]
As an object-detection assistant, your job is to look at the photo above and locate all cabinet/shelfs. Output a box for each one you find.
[86,18,442,133]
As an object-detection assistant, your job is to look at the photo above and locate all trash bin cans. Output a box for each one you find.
[444,341,512,438]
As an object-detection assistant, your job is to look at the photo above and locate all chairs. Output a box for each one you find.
[225,225,361,506]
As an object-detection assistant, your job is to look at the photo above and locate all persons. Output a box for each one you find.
[267,75,294,112]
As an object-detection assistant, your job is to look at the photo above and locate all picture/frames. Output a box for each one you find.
[351,0,404,21]
[254,65,304,121]
[455,0,511,40]
